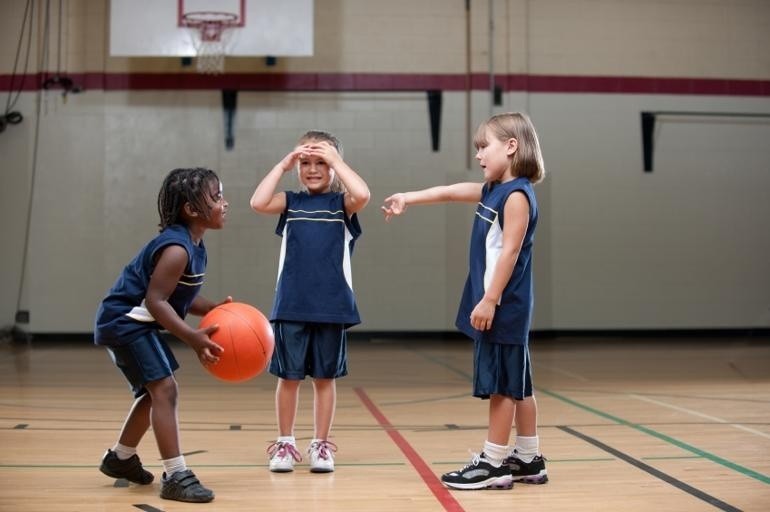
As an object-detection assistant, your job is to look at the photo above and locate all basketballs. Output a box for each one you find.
[198,302,275,380]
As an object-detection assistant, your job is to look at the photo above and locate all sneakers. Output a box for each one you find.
[441,455,517,491]
[160,470,215,504]
[266,439,301,472]
[502,451,549,483]
[307,439,338,472]
[99,449,155,484]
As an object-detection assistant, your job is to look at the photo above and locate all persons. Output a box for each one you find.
[94,167,234,504]
[380,112,551,491]
[249,130,371,472]
